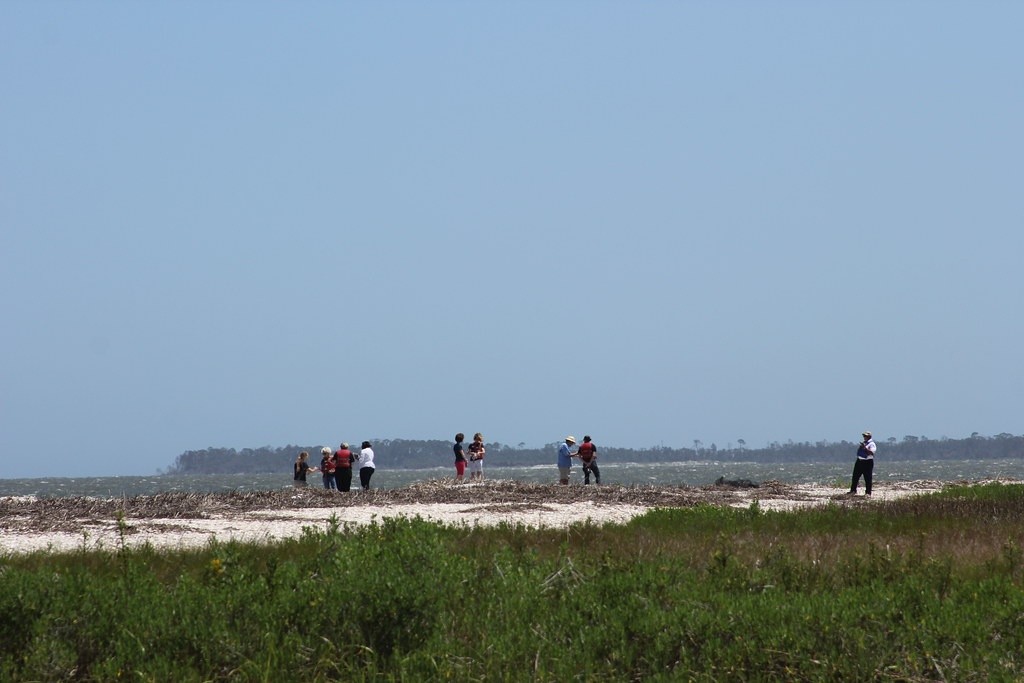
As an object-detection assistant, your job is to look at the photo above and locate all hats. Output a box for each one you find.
[583,435,591,441]
[565,436,576,443]
[340,442,348,448]
[862,431,872,436]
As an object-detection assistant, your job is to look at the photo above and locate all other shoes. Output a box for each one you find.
[864,494,871,497]
[847,491,857,494]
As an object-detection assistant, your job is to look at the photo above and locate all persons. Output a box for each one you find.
[466,433,485,480]
[320,447,336,489]
[354,441,375,491]
[331,442,355,492]
[846,431,877,496]
[293,451,318,487]
[556,436,582,485]
[577,436,600,485]
[453,433,468,480]
